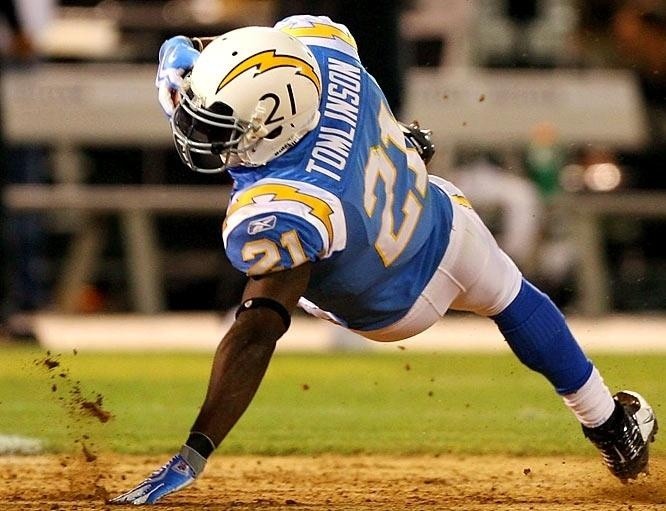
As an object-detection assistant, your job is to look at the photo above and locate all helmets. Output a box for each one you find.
[166,27,323,173]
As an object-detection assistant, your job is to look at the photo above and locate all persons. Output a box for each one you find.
[2,0,666,326]
[102,14,660,507]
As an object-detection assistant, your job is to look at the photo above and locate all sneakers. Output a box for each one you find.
[591,391,657,483]
[398,117,436,164]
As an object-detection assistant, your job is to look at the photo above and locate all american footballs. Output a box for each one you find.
[172,67,194,108]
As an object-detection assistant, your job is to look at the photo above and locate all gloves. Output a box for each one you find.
[108,443,206,506]
[155,34,197,117]
